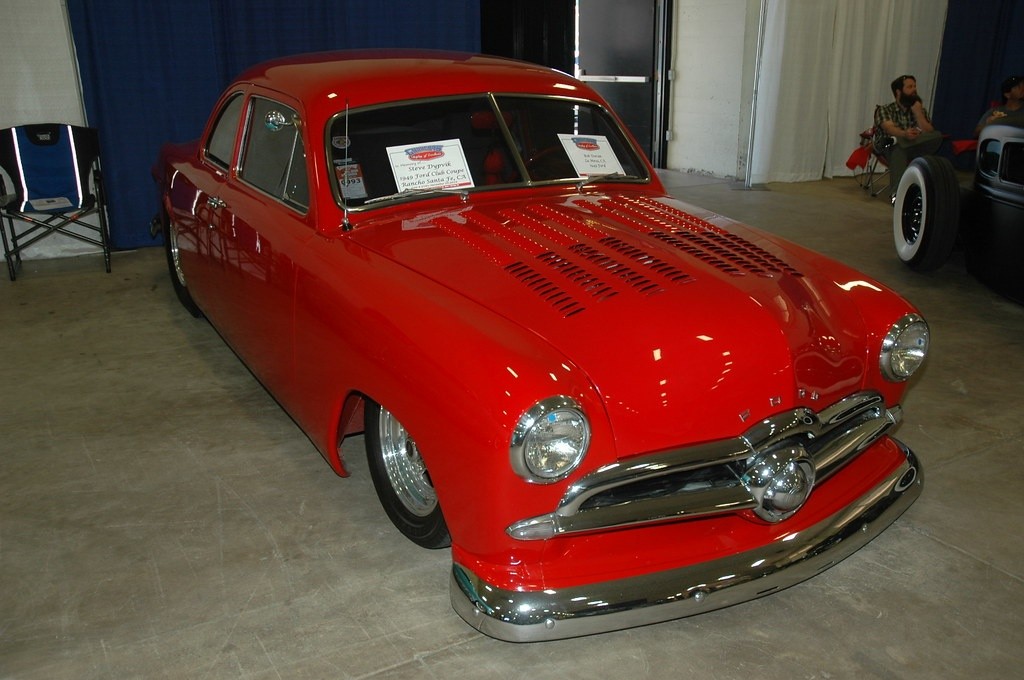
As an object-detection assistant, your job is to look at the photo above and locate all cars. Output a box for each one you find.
[152,51,930,643]
[893,106,1024,273]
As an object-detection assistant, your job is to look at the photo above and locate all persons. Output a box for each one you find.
[951,75,1024,174]
[875,75,942,205]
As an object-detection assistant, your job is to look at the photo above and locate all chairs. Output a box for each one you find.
[845,104,952,196]
[0,123,111,281]
[954,100,1005,155]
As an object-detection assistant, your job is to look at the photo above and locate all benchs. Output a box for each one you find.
[330,126,528,208]
